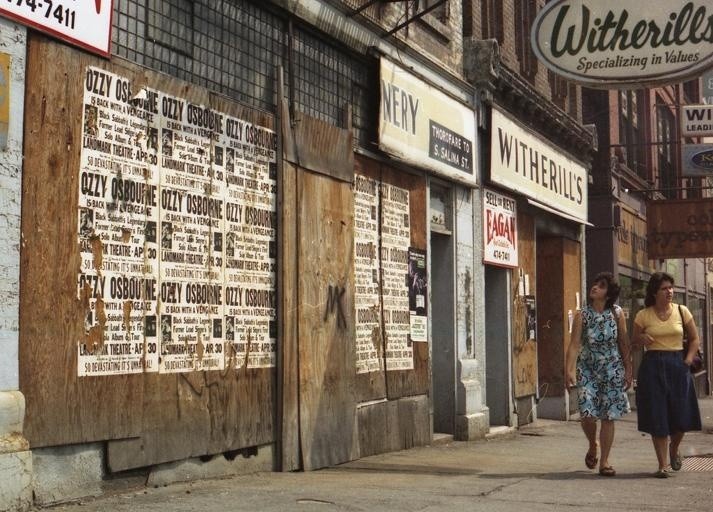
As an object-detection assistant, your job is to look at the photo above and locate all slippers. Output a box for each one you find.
[598,465,616,475]
[583,450,598,470]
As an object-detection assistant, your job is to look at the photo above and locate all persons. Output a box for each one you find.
[564,270,635,479]
[629,272,701,478]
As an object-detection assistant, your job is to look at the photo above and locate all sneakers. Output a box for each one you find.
[654,468,669,477]
[669,441,682,470]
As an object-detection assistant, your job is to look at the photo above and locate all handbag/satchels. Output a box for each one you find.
[676,304,703,374]
